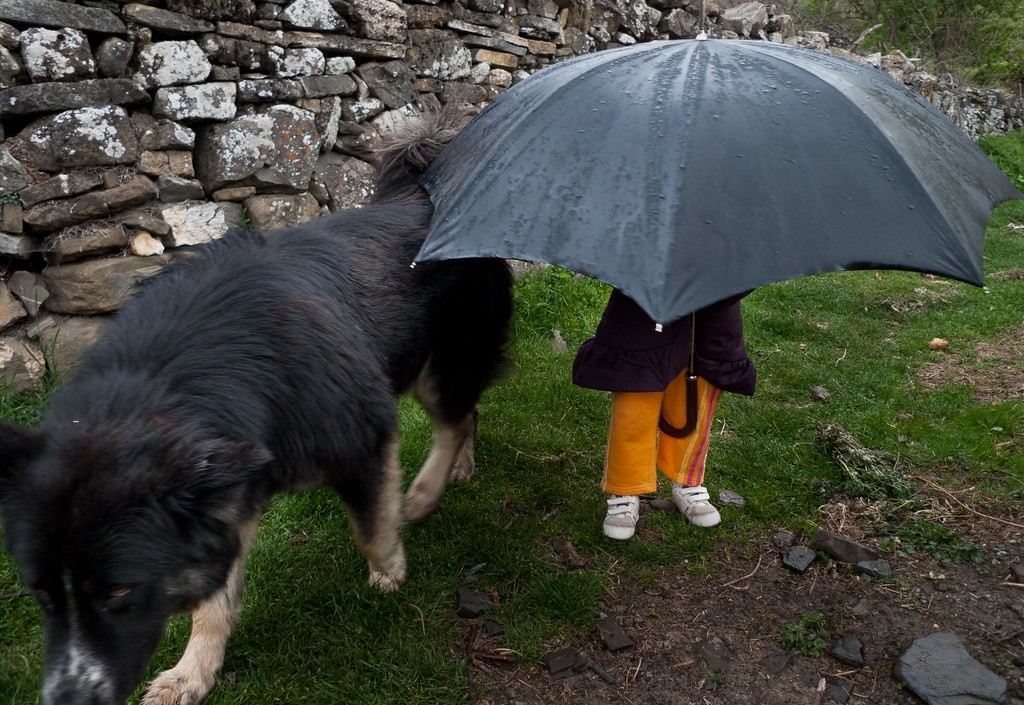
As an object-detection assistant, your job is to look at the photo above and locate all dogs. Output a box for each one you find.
[0,93,516,705]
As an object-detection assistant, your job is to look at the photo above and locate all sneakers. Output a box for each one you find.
[672,484,721,527]
[603,493,639,540]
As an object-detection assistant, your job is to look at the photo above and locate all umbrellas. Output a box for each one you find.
[405,0,1024,333]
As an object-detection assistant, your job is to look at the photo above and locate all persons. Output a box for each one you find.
[571,275,758,540]
